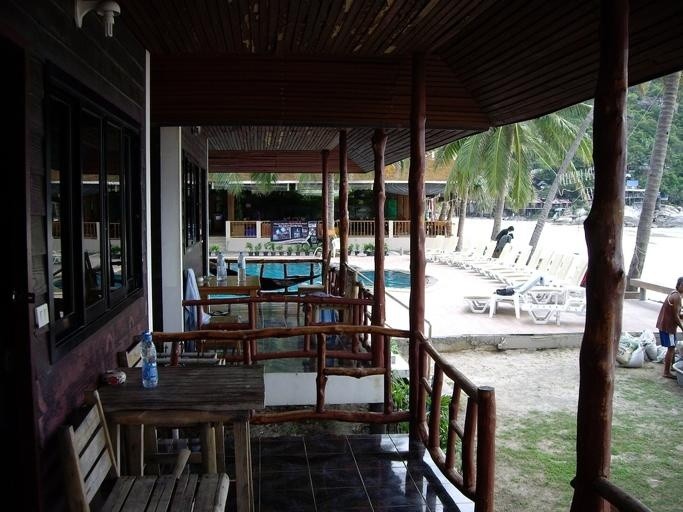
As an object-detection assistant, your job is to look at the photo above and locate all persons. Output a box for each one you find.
[495,226,514,245]
[492,233,513,258]
[656,277,683,379]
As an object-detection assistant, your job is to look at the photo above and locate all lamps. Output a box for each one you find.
[74,0,122,38]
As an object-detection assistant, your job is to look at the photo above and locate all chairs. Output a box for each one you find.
[400,235,586,326]
[297,250,332,317]
[186,268,241,366]
[118,336,143,368]
[84,250,122,301]
[61,391,231,512]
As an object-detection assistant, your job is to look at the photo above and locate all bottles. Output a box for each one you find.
[216,251,228,282]
[236,251,247,283]
[140,332,158,389]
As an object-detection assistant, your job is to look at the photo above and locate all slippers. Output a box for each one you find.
[662,375,676,379]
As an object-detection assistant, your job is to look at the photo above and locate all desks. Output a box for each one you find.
[96,365,266,512]
[199,276,262,356]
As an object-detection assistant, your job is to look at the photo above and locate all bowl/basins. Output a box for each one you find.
[671,359,683,383]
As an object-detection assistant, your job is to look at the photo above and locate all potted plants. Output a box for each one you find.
[246,241,388,256]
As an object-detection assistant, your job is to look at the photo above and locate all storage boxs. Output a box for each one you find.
[672,359,683,388]
